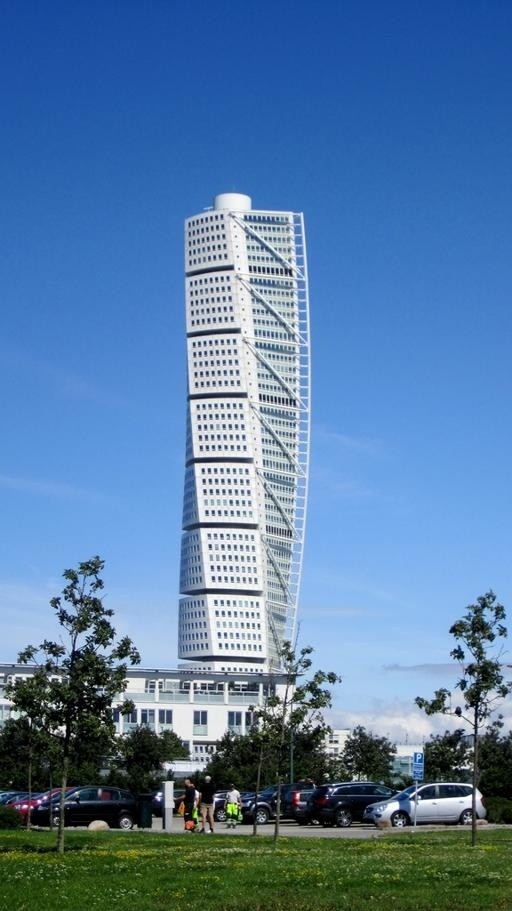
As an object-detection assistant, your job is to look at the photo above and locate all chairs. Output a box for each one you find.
[101,792,112,801]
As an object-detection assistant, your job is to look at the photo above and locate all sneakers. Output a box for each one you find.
[189,828,214,833]
[226,824,236,829]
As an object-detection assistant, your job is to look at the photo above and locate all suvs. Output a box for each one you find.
[149,782,319,825]
[29,785,139,830]
[305,781,403,828]
[361,782,488,827]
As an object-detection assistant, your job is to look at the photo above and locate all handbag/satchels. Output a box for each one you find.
[238,808,243,821]
[185,820,194,830]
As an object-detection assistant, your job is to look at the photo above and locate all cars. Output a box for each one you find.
[0,786,112,823]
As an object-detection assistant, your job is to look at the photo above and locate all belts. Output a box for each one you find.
[227,802,237,805]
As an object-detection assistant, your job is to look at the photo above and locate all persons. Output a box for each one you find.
[199,776,215,834]
[224,784,243,829]
[172,779,195,832]
[190,784,199,832]
